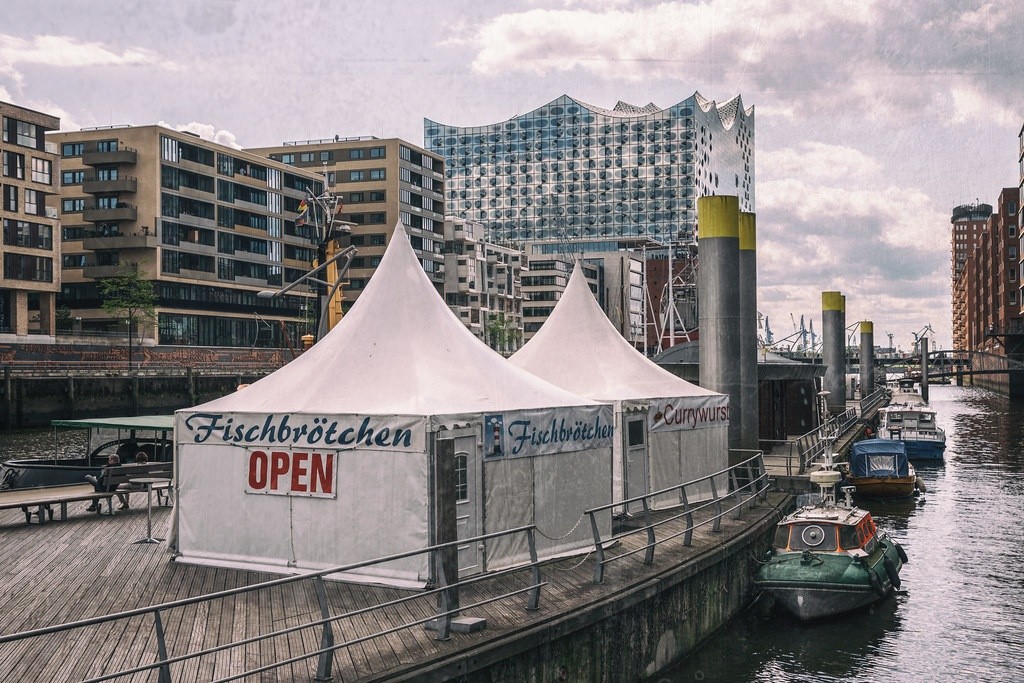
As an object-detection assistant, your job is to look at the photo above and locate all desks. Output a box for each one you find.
[129,477,171,544]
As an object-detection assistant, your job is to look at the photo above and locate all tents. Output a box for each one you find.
[164,215,731,587]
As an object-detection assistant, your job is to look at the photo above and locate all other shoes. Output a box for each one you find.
[87,504,99,511]
[118,503,129,509]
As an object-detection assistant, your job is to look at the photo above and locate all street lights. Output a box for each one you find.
[256,245,359,343]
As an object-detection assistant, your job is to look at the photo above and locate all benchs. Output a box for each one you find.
[104,462,174,509]
[0,492,114,526]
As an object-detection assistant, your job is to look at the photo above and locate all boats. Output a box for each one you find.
[1,160,359,491]
[840,406,916,500]
[876,365,947,460]
[751,375,904,622]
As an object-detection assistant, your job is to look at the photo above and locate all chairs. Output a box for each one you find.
[119,441,172,461]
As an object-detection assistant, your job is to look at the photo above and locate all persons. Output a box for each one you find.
[85,454,126,511]
[115,452,150,509]
[866,427,876,439]
